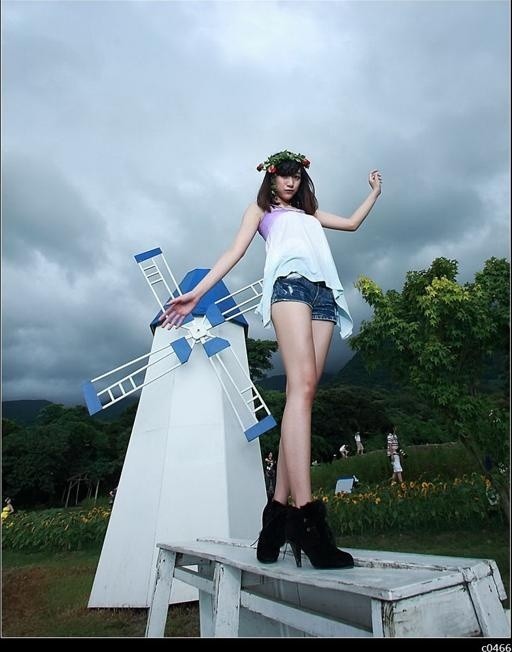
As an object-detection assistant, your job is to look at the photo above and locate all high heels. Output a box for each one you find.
[255,498,355,570]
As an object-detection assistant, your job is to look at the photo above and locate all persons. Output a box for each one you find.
[264,451,277,490]
[391,434,399,454]
[385,432,394,456]
[109,487,117,502]
[157,149,383,571]
[1,496,16,528]
[339,443,349,458]
[354,431,364,455]
[389,449,404,482]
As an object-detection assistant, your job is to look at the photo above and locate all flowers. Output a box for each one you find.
[256,150,310,173]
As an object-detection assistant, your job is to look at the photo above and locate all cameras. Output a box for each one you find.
[395,446,408,459]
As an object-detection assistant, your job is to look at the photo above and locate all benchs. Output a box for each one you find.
[144,538,510,638]
